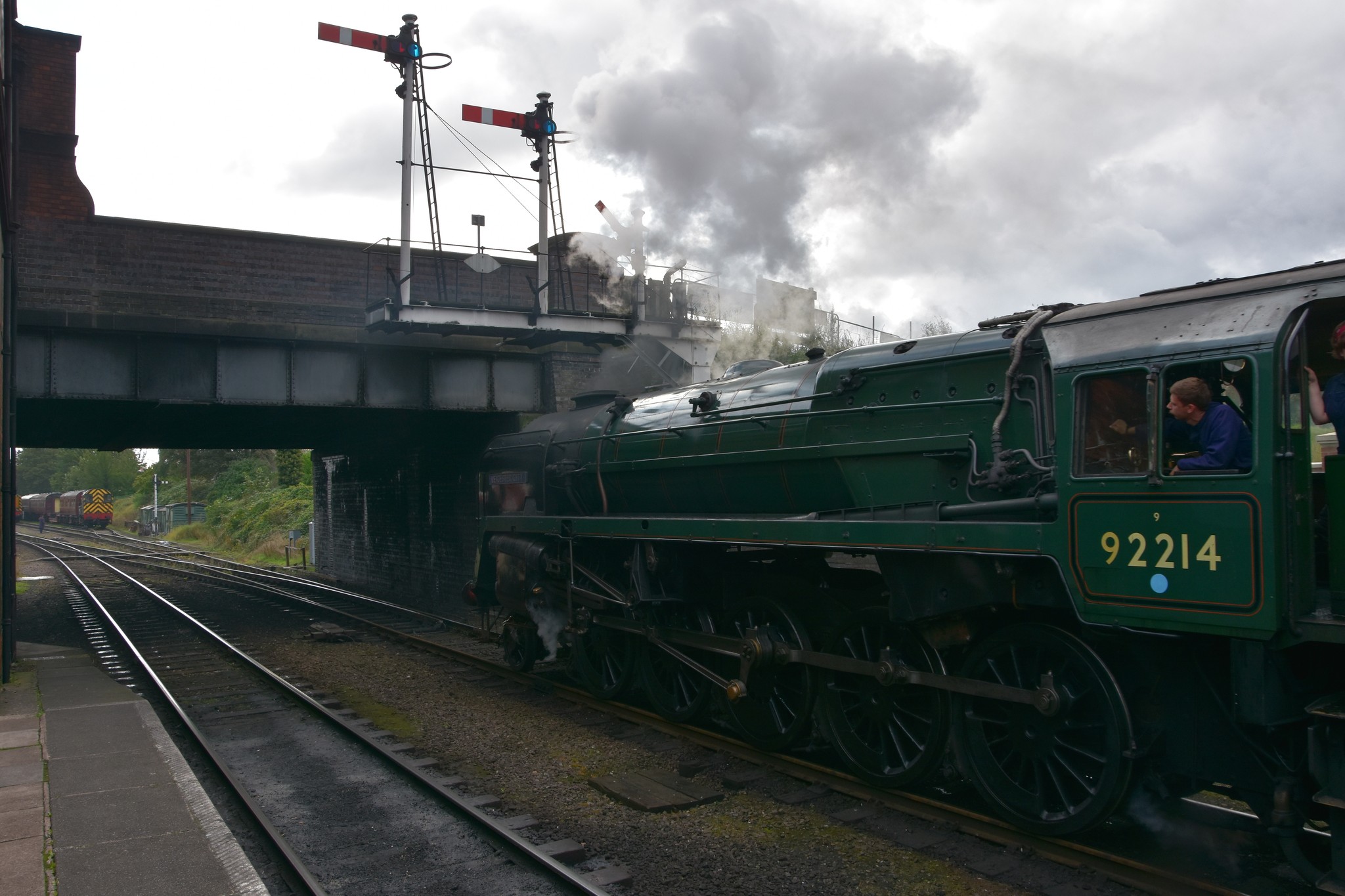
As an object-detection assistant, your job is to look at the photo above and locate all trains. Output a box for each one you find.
[464,257,1345,895]
[14,488,114,530]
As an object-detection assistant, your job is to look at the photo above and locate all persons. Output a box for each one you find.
[39,515,45,533]
[1304,325,1345,468]
[1109,377,1252,475]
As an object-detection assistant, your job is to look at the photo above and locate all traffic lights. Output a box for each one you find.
[385,25,422,66]
[523,103,556,143]
